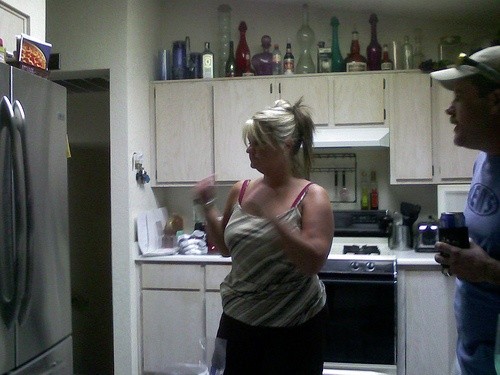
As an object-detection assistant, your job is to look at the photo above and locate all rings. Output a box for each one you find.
[445,269,451,278]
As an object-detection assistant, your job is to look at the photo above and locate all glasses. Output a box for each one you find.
[456,52,500,81]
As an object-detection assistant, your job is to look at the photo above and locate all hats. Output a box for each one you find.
[429,45,500,92]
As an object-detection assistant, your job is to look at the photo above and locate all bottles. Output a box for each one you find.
[346,30,368,71]
[401,35,415,69]
[158,49,171,80]
[190,52,203,79]
[296,3,316,74]
[329,15,347,72]
[200,41,213,78]
[218,2,231,78]
[360,170,370,209]
[271,44,283,75]
[380,43,394,70]
[172,41,187,80]
[409,26,426,70]
[226,41,236,77]
[370,170,378,210]
[236,20,251,77]
[367,12,382,70]
[439,36,460,69]
[283,41,295,74]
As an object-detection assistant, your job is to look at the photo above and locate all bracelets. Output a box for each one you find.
[200,198,216,210]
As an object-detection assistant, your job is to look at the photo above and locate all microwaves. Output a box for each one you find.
[319,261,398,373]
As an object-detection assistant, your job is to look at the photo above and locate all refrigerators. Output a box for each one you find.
[0,60,74,375]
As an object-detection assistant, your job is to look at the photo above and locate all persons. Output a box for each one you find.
[434,45,500,375]
[191,96,336,375]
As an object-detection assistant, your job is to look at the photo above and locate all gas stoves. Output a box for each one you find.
[343,244,382,256]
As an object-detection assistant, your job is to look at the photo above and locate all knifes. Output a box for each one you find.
[334,171,338,202]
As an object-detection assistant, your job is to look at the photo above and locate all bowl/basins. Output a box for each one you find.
[388,225,413,252]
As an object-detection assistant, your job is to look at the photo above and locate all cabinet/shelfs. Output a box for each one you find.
[150,75,276,187]
[389,70,433,184]
[406,270,457,375]
[276,71,390,127]
[310,156,357,203]
[434,80,482,184]
[205,264,234,375]
[141,263,205,375]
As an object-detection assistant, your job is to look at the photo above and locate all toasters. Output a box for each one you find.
[416,220,441,254]
